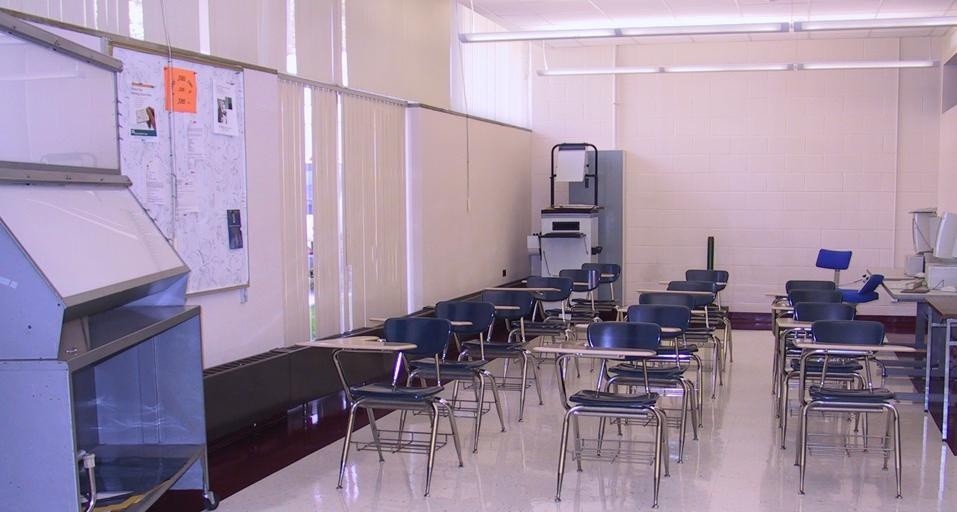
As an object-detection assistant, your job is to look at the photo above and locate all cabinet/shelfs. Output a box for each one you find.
[0,302,220,511]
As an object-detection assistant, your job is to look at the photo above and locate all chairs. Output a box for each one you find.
[770,273,904,498]
[334,263,733,510]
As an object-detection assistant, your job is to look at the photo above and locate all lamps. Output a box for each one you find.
[534,33,942,79]
[456,1,956,47]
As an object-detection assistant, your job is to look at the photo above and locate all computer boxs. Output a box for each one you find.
[904,255,924,273]
[925,257,957,289]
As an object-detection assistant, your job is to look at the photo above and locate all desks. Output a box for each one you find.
[864,267,957,444]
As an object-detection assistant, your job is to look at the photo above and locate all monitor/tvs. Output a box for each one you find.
[933,212,957,262]
[912,212,938,254]
